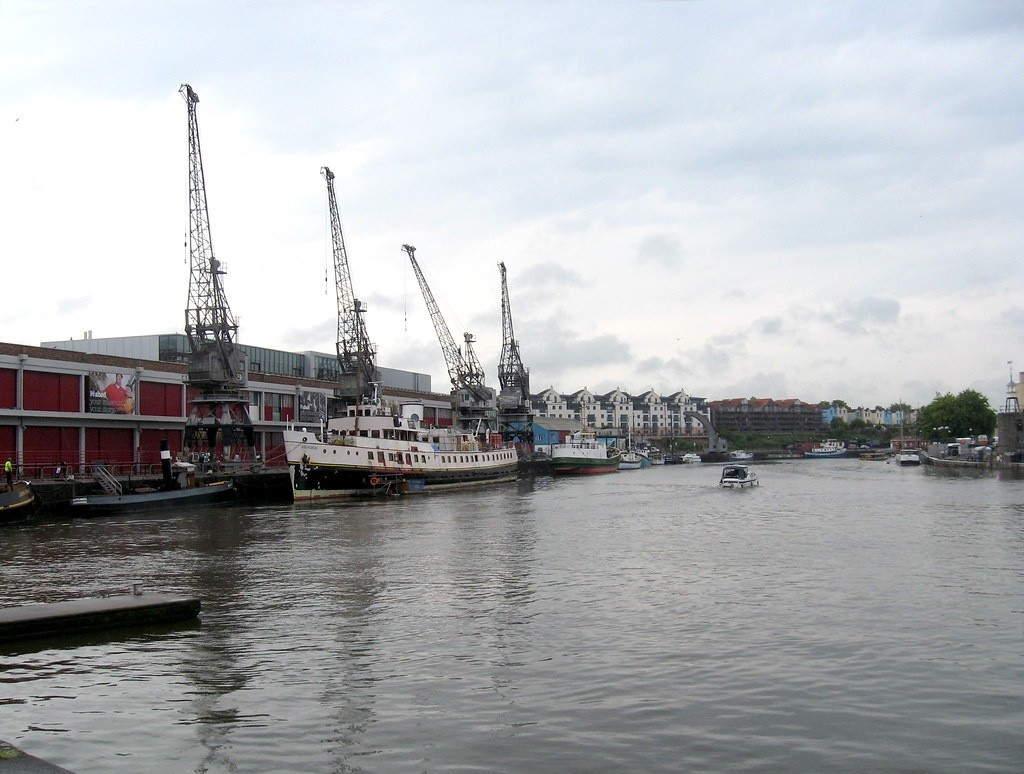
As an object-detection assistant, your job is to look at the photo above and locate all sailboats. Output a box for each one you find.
[893,398,919,466]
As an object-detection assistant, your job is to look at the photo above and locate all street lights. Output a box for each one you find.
[933,426,951,457]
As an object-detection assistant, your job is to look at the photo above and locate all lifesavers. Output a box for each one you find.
[370,477,378,486]
[960,439,965,444]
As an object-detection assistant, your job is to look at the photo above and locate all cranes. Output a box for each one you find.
[497,261,535,455]
[178,83,260,462]
[318,166,393,416]
[400,243,496,432]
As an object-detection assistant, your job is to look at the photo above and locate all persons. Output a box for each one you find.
[104,373,136,414]
[5,458,13,485]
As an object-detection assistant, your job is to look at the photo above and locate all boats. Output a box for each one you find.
[681,452,701,463]
[44,439,237,520]
[0,481,36,520]
[804,439,847,458]
[549,428,621,474]
[283,380,519,500]
[719,464,758,489]
[618,423,642,469]
[635,440,665,465]
[731,450,753,460]
[860,452,889,461]
